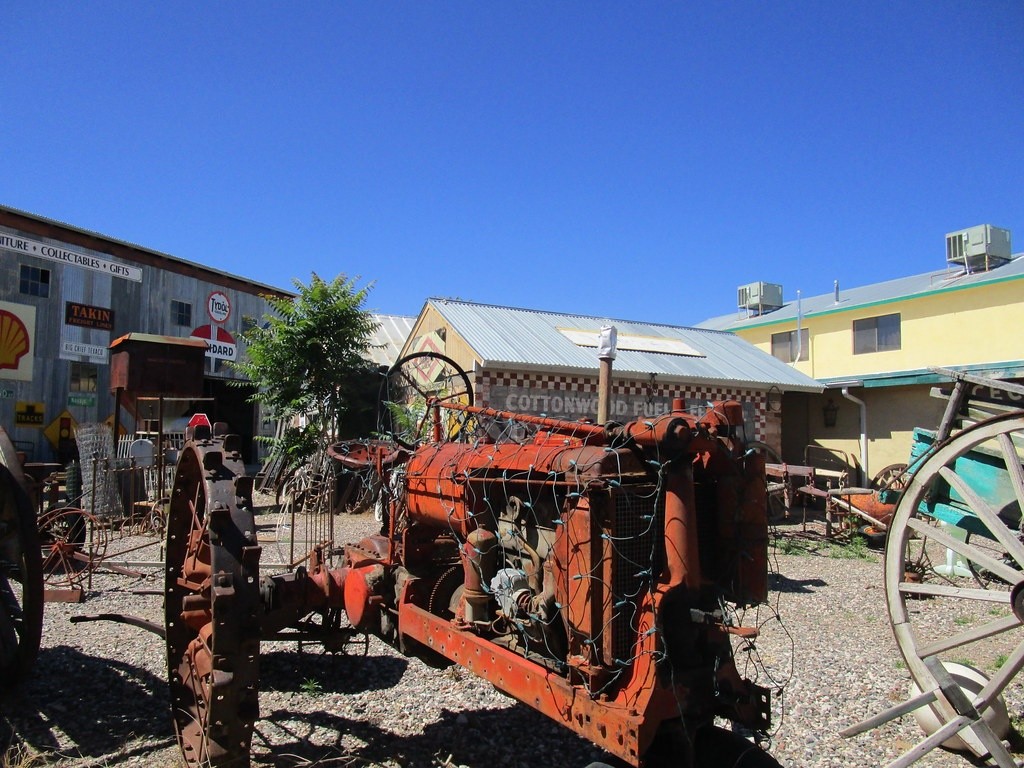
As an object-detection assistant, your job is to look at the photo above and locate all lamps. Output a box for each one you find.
[822,398,839,428]
